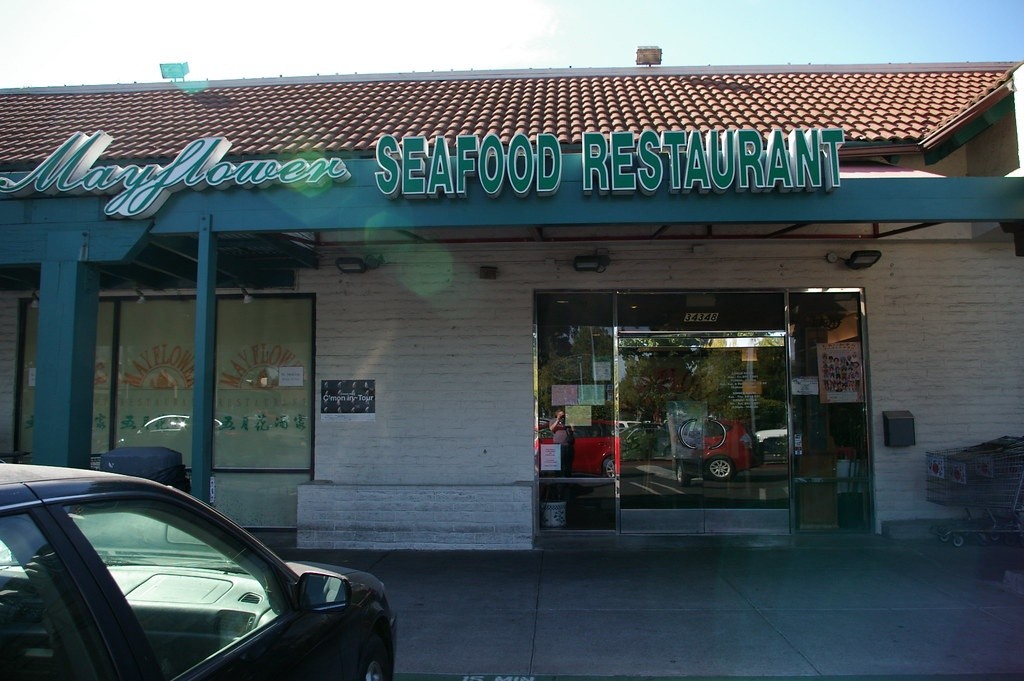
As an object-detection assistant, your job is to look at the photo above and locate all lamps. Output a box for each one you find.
[572,254,612,274]
[824,249,882,271]
[333,250,386,274]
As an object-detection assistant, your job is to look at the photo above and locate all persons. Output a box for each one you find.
[550,408,577,486]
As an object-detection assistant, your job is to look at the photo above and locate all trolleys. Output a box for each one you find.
[918,432,1024,548]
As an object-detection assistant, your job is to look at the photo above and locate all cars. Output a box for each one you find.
[112,414,243,451]
[533,411,789,484]
[0,460,397,681]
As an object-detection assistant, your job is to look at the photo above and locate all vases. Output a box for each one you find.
[541,498,567,530]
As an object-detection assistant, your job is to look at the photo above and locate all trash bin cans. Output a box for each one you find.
[99,445,190,495]
